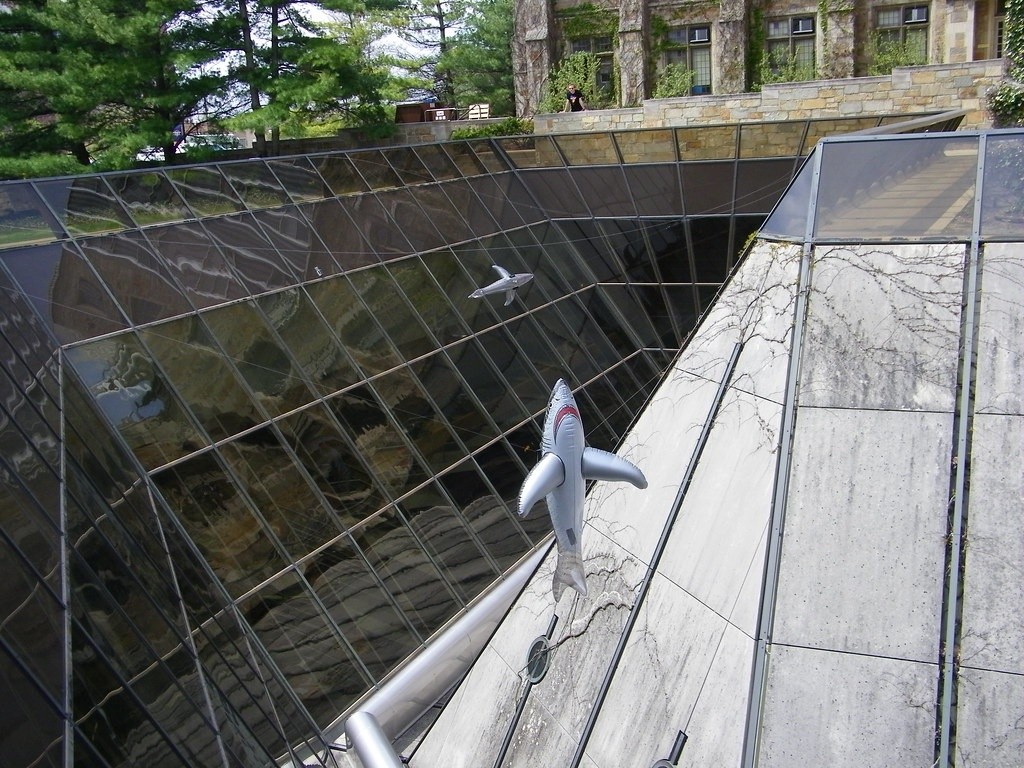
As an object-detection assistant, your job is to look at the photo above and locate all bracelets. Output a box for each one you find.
[583,108,586,111]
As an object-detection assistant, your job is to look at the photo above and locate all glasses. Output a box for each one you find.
[569,88,574,90]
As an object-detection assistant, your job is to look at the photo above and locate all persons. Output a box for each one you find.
[559,84,586,113]
